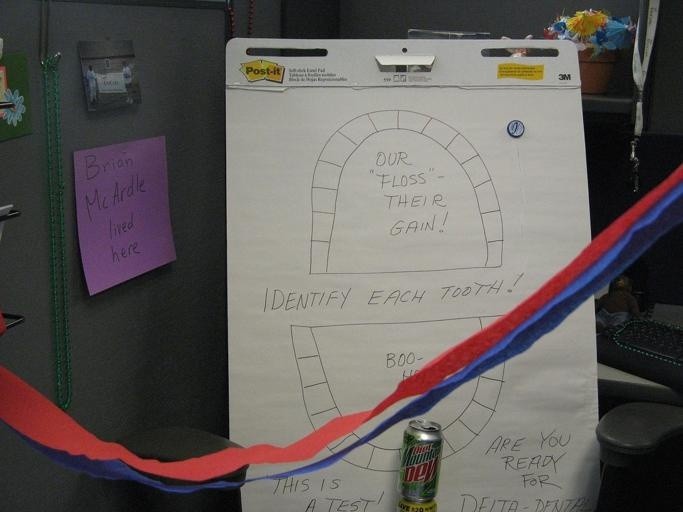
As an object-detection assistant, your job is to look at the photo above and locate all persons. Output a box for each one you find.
[122,59,134,105]
[86,63,98,102]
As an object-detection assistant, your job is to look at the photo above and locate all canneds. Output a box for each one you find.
[397,418,445,512]
